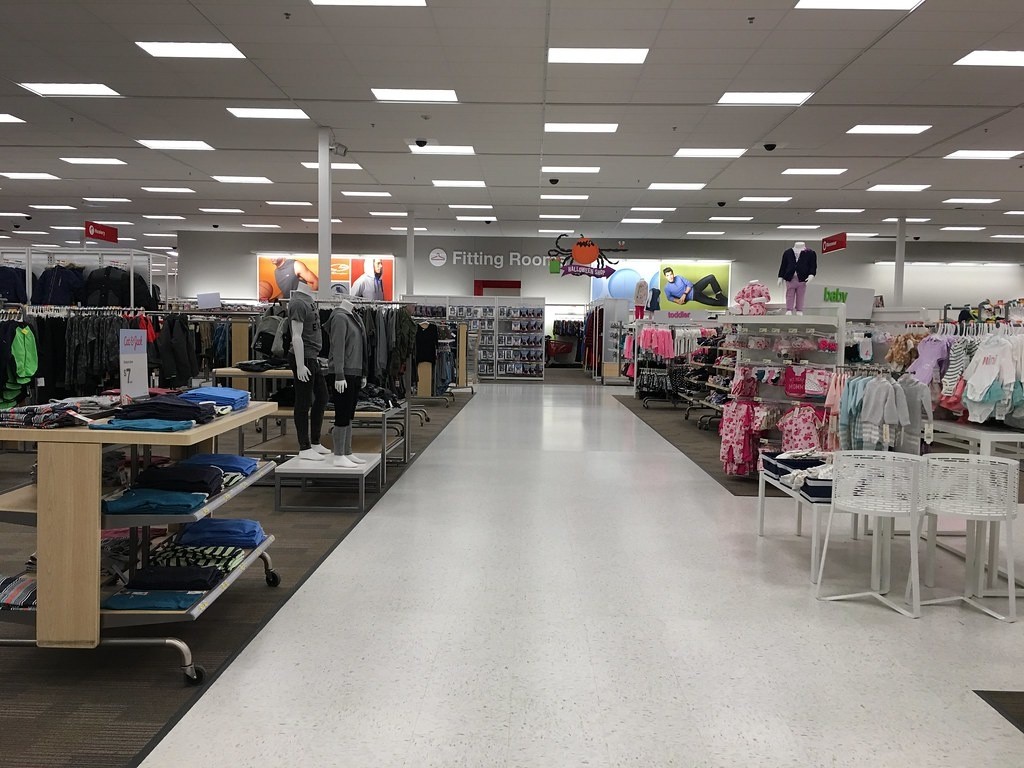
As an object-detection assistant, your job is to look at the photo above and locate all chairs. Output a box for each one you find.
[904,453,1018,623]
[815,451,927,619]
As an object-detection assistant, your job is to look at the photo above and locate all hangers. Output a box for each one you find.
[931,321,1024,341]
[0,303,146,323]
[110,260,130,272]
[903,323,931,335]
[833,363,914,382]
[169,311,182,316]
[53,259,66,267]
[2,257,25,269]
[319,299,404,313]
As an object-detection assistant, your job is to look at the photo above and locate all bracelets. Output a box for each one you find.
[683,293,687,295]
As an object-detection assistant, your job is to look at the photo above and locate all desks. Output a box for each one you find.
[275,453,382,511]
[265,400,408,483]
[211,366,293,455]
[758,452,999,589]
[921,415,1024,501]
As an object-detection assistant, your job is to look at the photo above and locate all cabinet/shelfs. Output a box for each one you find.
[398,293,545,380]
[586,298,628,386]
[1,402,278,684]
[677,344,737,411]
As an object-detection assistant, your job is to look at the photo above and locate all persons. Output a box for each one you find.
[663,267,727,306]
[633,278,649,324]
[778,242,817,316]
[327,298,366,468]
[734,280,771,315]
[267,257,318,299]
[350,258,384,301]
[288,282,332,461]
[646,285,661,323]
